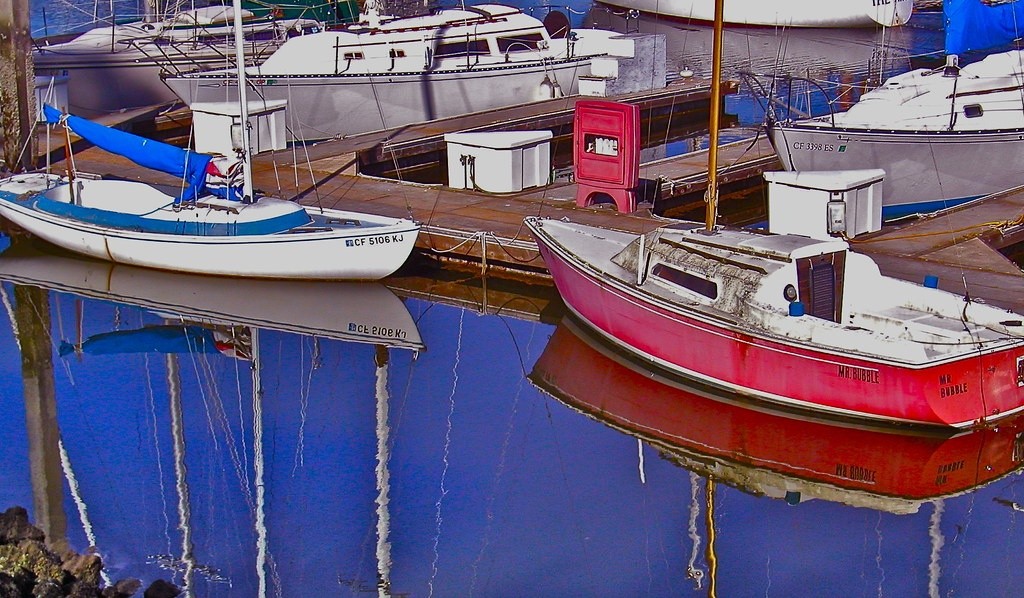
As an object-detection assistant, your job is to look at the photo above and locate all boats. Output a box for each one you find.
[526,314,1023,598]
[516,3,1023,431]
[162,2,636,158]
[759,48,1024,226]
[26,0,322,116]
[591,1,916,30]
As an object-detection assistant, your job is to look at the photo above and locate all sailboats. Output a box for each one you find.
[1,228,432,598]
[1,0,423,279]
